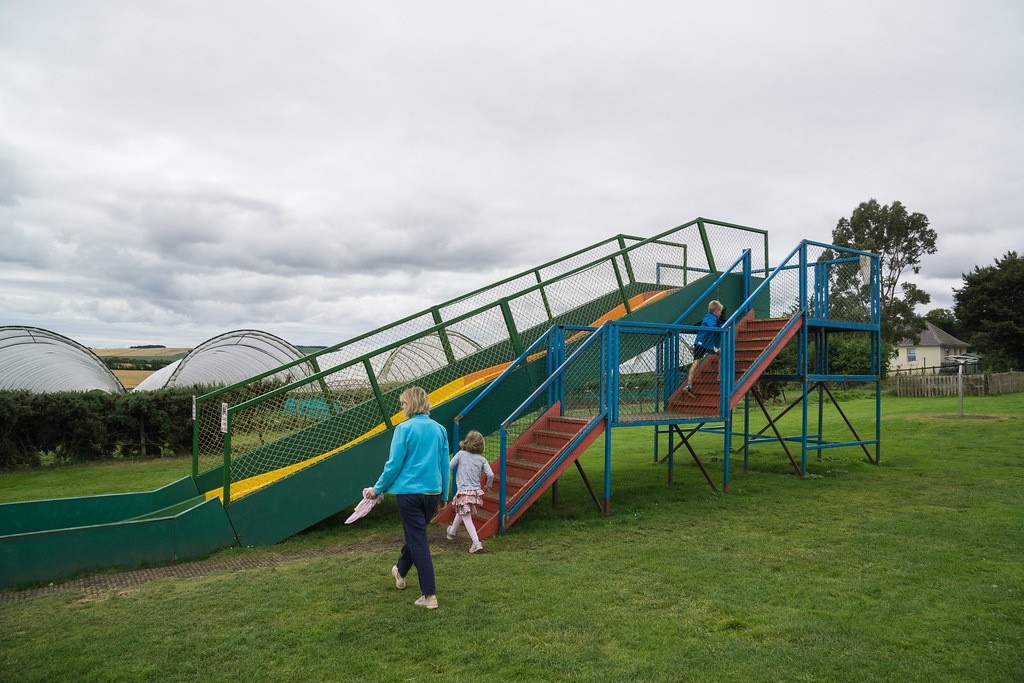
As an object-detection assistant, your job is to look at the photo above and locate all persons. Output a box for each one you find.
[365,386,450,610]
[683,300,724,399]
[446,431,494,553]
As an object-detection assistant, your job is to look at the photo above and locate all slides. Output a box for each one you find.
[205,286,676,507]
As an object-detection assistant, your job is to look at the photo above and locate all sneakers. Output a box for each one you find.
[469,542,483,553]
[414,595,438,608]
[446,525,457,540]
[682,385,695,398]
[392,565,406,589]
